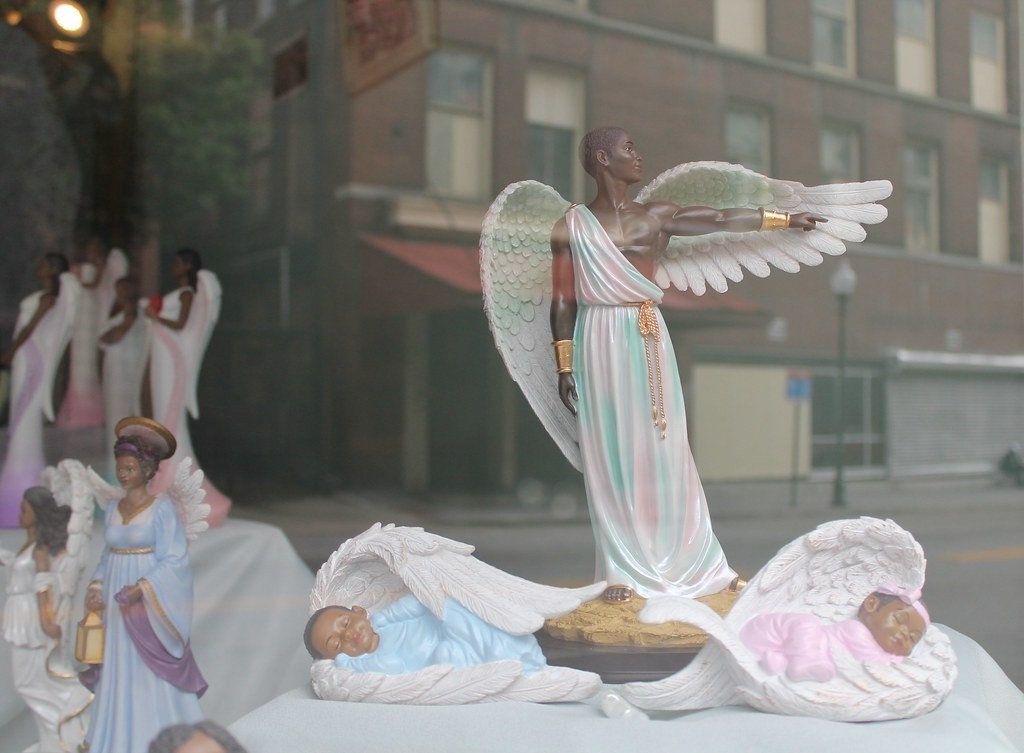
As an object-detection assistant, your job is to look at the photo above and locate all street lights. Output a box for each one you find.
[823,254,858,509]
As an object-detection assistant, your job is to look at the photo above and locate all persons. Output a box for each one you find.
[548,129,829,607]
[150,720,248,752]
[2,233,237,527]
[302,593,551,671]
[85,436,207,753]
[734,590,928,689]
[6,483,98,752]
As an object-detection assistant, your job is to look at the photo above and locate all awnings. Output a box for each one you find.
[358,229,764,317]
